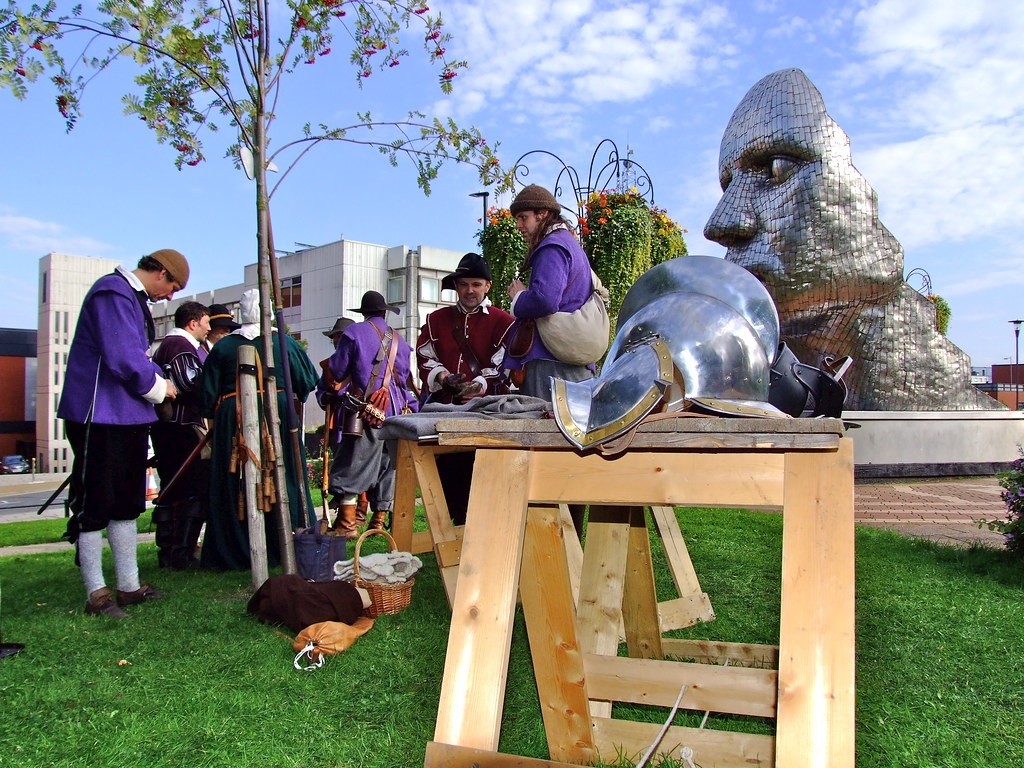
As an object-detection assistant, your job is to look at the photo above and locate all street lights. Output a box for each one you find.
[468,191,491,231]
[1008,320,1024,410]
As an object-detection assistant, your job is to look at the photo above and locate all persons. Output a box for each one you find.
[199,305,242,364]
[509,184,596,403]
[56,249,189,621]
[703,68,1011,411]
[326,290,423,537]
[416,253,516,408]
[150,301,211,570]
[196,287,321,572]
[316,318,368,525]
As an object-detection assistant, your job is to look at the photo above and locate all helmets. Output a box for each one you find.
[768,340,845,420]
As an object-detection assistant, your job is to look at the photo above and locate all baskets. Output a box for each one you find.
[348,529,415,618]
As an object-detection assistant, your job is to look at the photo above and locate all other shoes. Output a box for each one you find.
[157,548,204,572]
[85,587,128,618]
[115,582,162,607]
[0,643,25,659]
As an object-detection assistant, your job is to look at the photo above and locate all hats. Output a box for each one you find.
[509,183,561,218]
[151,248,190,290]
[350,291,400,316]
[440,253,491,291]
[207,303,242,333]
[322,317,355,339]
[238,289,276,324]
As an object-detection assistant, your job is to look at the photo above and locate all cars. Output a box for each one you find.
[0,455,30,475]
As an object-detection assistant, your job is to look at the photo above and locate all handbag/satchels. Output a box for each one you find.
[535,293,610,366]
[295,518,346,581]
[293,617,376,671]
[246,575,363,634]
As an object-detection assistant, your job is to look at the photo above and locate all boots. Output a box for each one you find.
[356,500,368,526]
[366,511,386,537]
[325,505,357,538]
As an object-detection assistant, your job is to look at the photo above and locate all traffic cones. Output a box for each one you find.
[145,467,159,501]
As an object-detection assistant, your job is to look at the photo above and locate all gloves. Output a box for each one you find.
[442,374,471,394]
[458,381,483,399]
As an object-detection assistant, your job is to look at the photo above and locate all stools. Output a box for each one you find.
[412,408,862,768]
[378,394,716,638]
